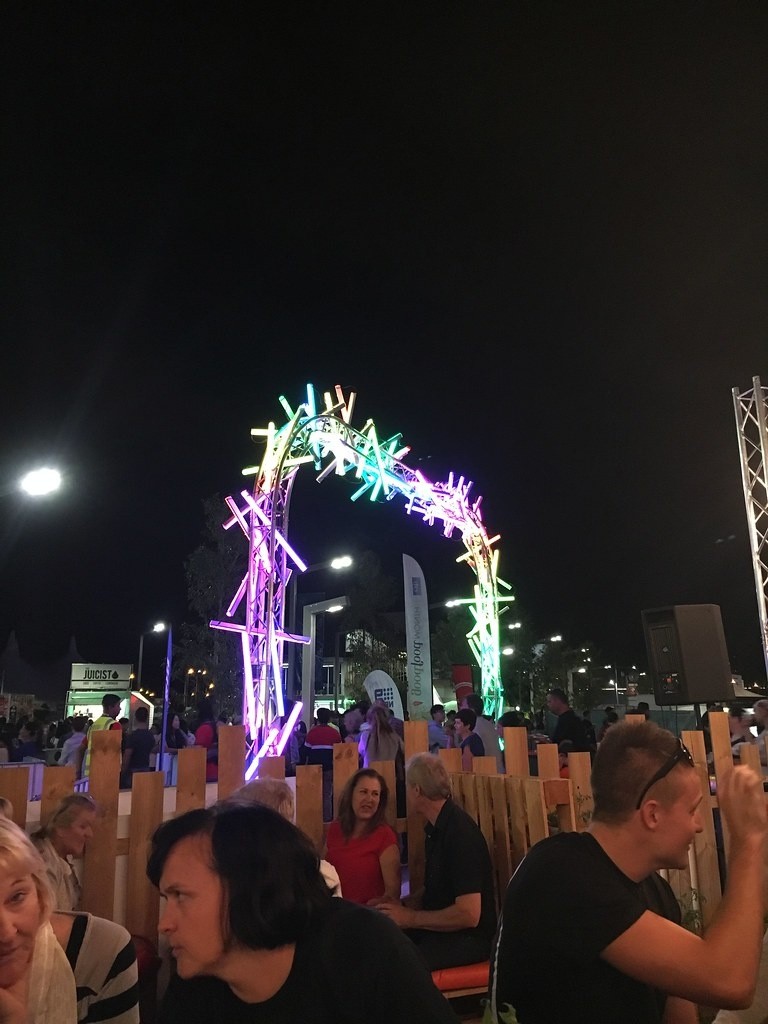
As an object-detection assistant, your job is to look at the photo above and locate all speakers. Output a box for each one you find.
[641,604,736,706]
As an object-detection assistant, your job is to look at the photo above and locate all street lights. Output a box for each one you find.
[299,595,352,739]
[528,633,563,725]
[286,552,353,702]
[137,621,167,689]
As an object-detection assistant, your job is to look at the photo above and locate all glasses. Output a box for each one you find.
[636,737,694,810]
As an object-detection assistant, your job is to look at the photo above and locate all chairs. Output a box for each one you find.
[430,833,518,998]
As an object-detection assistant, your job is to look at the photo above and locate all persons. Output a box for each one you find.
[0,689,652,817]
[484,720,768,1024]
[147,800,456,1024]
[0,793,140,1024]
[218,752,498,973]
